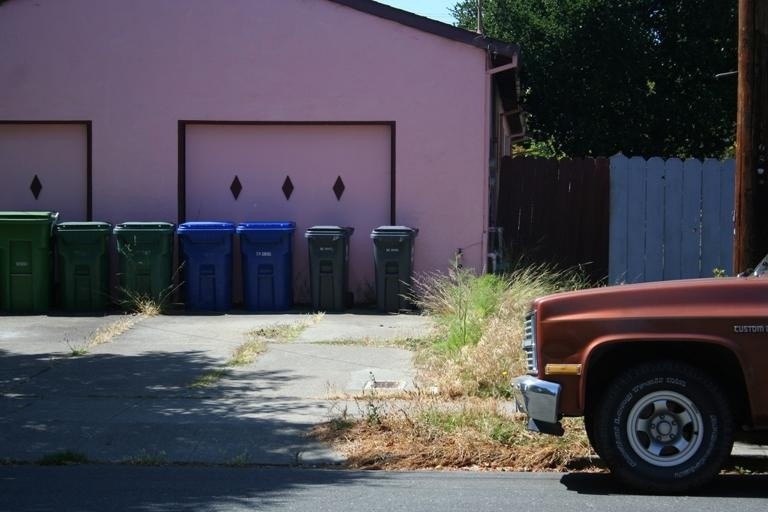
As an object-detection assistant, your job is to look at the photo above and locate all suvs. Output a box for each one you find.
[511,251,768,492]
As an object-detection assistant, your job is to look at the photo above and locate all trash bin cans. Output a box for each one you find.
[0,212,56,315]
[304,225,354,312]
[113,222,176,313]
[178,220,236,313]
[236,219,299,312]
[55,221,113,315]
[370,226,419,314]
